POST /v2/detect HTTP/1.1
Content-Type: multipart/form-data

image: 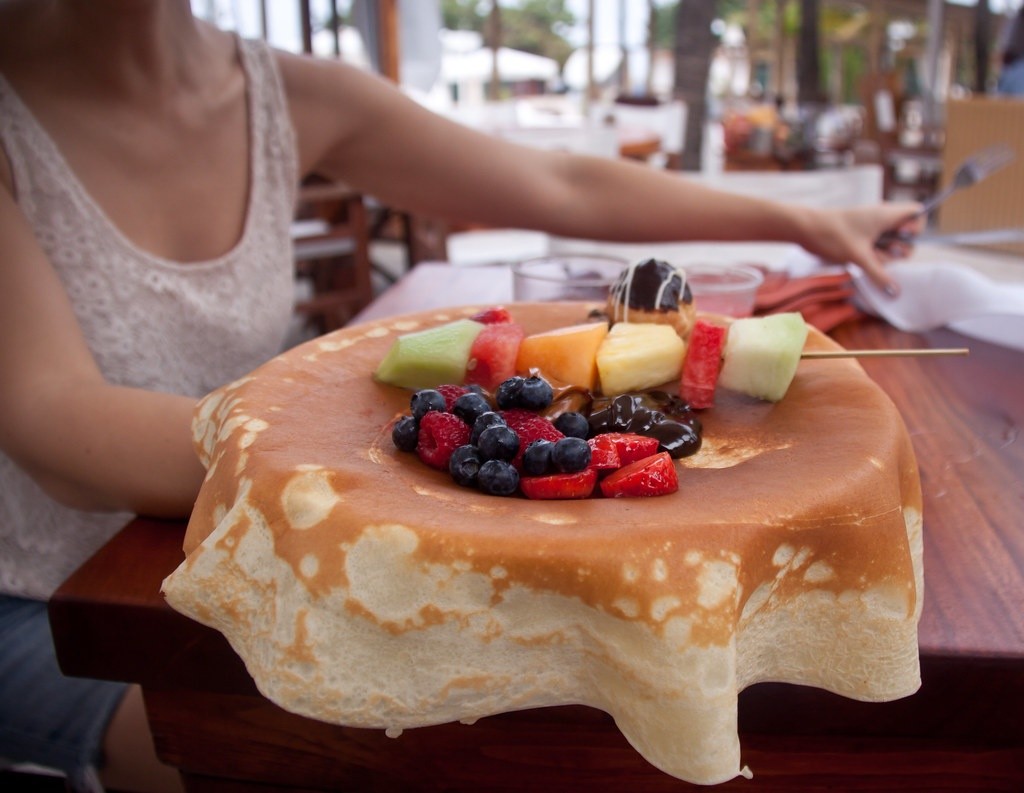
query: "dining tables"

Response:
[46,253,1024,793]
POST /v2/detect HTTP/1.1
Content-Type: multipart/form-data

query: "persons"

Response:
[2,0,930,793]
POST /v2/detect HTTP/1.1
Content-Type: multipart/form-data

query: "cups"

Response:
[510,252,635,301]
[687,260,766,319]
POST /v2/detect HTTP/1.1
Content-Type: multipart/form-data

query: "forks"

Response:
[890,152,1022,233]
